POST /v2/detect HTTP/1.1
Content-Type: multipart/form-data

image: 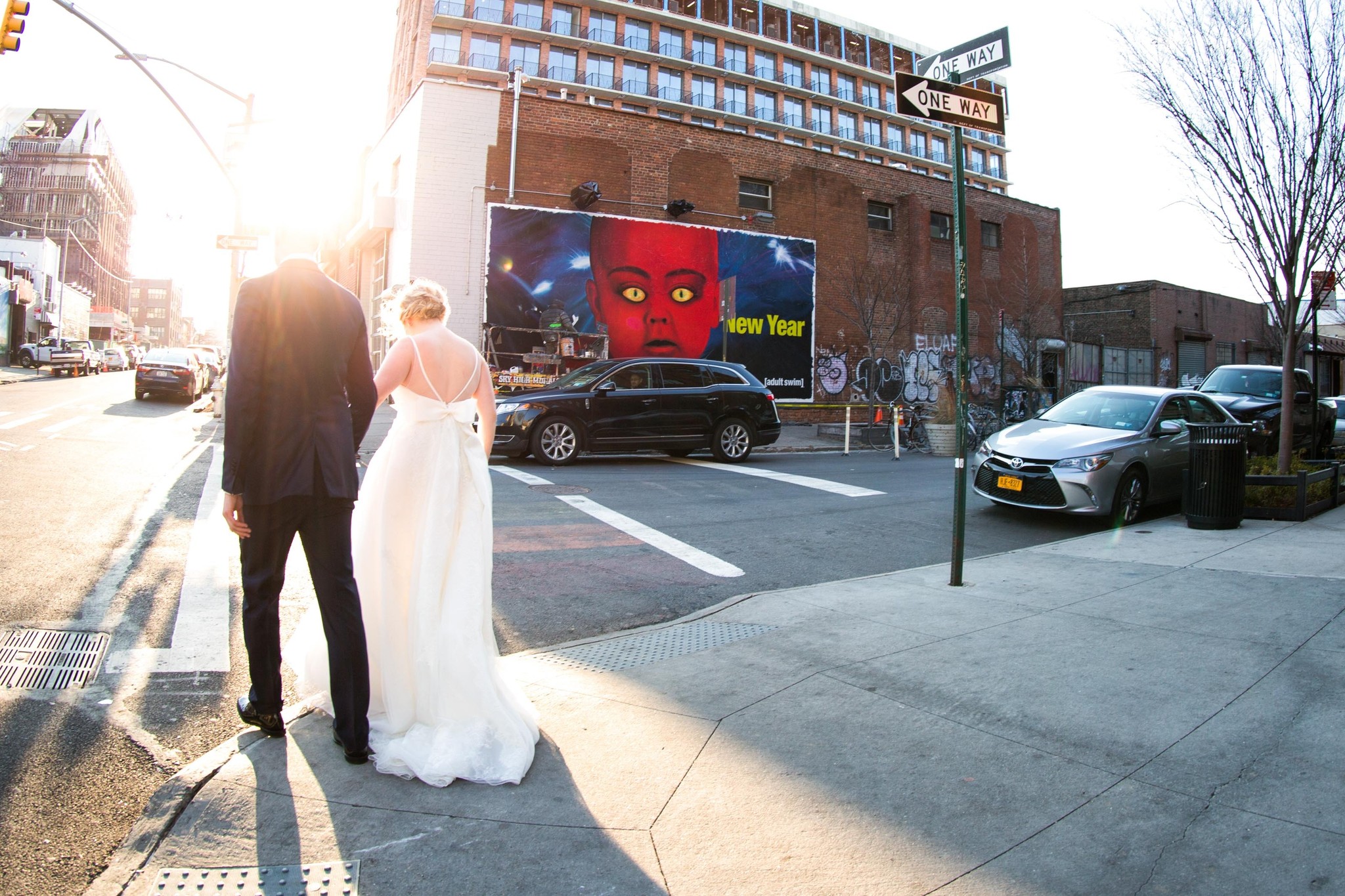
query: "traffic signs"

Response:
[894,71,1006,137]
[915,26,1012,86]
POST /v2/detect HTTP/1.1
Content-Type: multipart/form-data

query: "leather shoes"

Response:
[332,718,369,763]
[236,697,287,738]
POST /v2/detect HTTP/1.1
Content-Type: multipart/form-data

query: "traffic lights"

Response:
[0,0,30,54]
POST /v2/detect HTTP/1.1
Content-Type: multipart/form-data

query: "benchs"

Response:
[1129,399,1209,427]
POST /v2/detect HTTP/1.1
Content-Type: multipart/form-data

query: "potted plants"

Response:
[924,383,956,457]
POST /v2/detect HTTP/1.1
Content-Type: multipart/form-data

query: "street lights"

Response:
[113,52,256,349]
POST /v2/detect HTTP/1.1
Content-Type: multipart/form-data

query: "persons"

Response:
[345,280,542,786]
[585,215,723,361]
[221,258,376,771]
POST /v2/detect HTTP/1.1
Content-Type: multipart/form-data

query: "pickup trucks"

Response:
[1193,364,1337,457]
[51,340,101,376]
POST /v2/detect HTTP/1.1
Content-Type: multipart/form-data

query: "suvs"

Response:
[16,336,81,369]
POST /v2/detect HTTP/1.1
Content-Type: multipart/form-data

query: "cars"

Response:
[970,385,1248,528]
[471,357,783,464]
[186,345,227,405]
[94,341,147,373]
[135,347,205,405]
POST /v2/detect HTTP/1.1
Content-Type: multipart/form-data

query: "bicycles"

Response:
[867,400,1008,454]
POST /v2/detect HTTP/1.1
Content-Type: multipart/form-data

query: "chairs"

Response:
[1098,399,1129,429]
[1139,399,1179,429]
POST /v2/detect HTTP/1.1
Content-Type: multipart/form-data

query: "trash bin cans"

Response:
[1184,422,1253,531]
